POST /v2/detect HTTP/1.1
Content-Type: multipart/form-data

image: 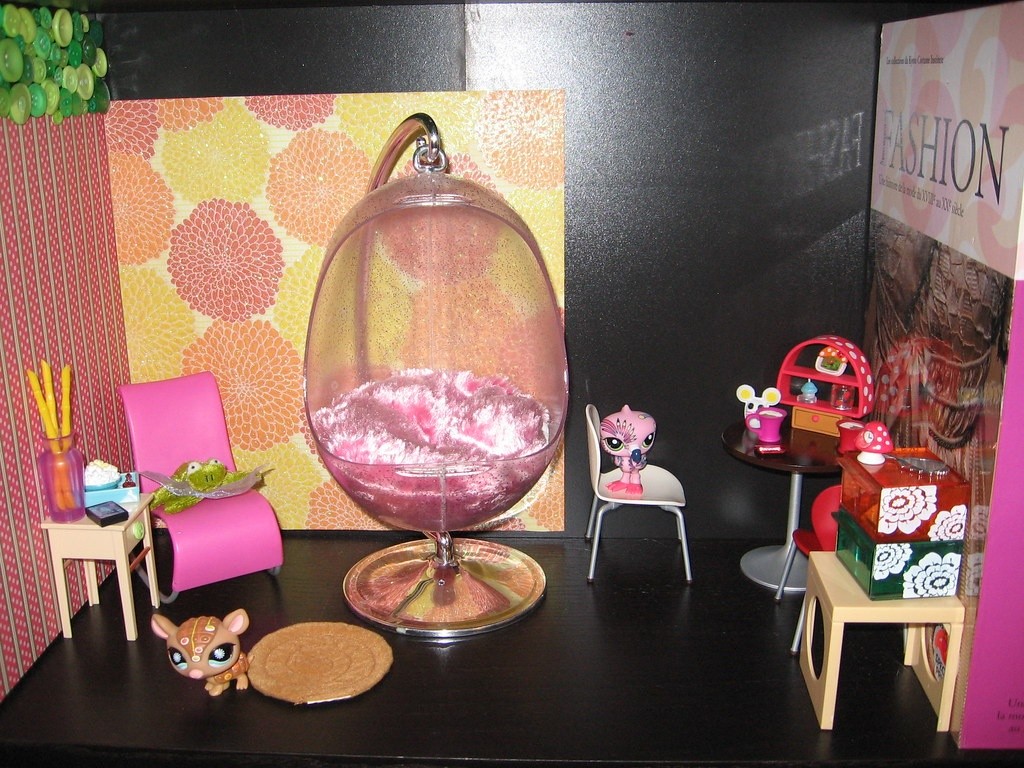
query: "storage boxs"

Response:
[790,406,851,437]
[830,446,972,601]
[82,501,129,528]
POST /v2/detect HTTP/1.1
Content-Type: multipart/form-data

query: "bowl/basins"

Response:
[83,475,122,493]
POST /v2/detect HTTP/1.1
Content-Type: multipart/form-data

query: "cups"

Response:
[745,407,788,443]
[829,383,855,411]
[835,418,867,455]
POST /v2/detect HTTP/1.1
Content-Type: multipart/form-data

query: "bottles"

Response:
[800,381,818,403]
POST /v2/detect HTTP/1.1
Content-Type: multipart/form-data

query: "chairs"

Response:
[120,370,286,605]
[774,482,844,656]
[584,405,694,585]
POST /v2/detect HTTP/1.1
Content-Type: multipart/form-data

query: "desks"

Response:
[721,420,842,593]
[39,493,160,642]
[797,550,968,733]
